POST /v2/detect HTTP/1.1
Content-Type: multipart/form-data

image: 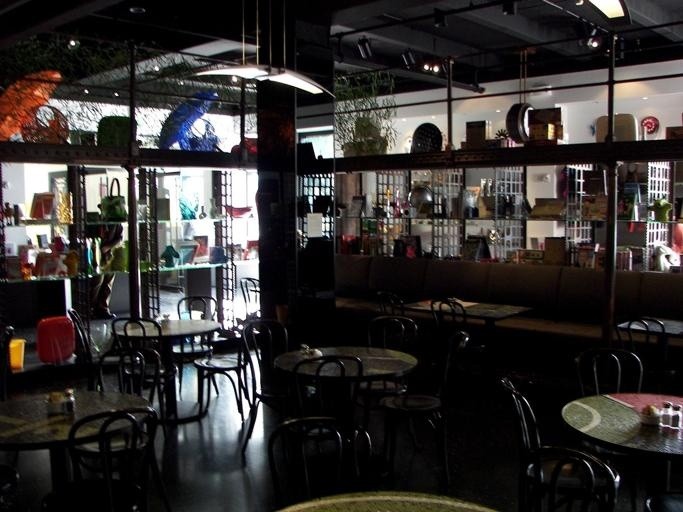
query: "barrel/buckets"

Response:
[10,337,26,371]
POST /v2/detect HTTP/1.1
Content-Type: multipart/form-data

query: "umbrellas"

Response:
[0,69,72,150]
[157,90,224,154]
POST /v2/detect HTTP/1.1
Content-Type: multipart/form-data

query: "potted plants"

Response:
[326,69,397,157]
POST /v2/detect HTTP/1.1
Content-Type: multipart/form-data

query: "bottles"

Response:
[640,401,682,430]
[48,389,76,415]
[299,344,310,361]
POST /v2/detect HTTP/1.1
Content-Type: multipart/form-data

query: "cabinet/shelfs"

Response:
[336,139,682,272]
[0,139,258,285]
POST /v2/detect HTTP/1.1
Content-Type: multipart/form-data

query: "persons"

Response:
[85,212,116,321]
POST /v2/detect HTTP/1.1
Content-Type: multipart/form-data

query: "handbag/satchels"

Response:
[101,195,128,223]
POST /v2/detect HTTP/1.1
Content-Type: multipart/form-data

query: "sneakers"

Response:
[91,308,117,321]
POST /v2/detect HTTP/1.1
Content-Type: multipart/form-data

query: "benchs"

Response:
[333,252,682,390]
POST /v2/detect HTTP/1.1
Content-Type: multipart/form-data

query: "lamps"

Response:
[190,1,336,102]
